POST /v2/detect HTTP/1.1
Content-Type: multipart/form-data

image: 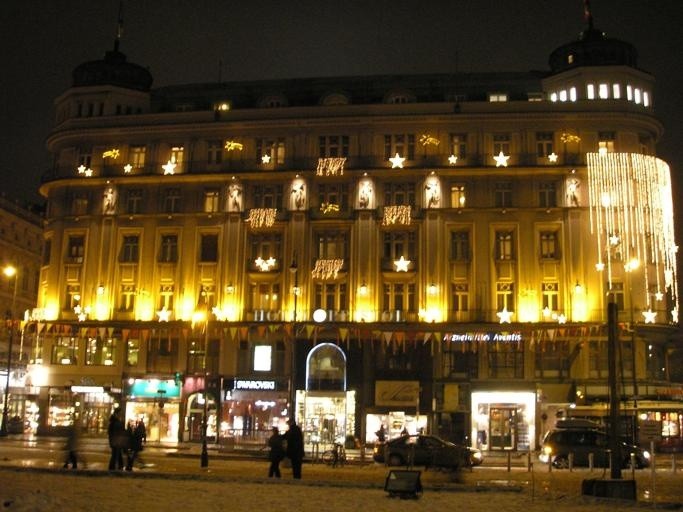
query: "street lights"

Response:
[0,256,20,437]
[195,289,211,468]
[289,248,300,421]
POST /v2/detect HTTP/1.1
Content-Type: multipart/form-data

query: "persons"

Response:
[400,427,409,437]
[108,408,147,472]
[567,177,580,208]
[360,182,371,209]
[281,419,305,479]
[61,424,77,474]
[104,188,114,214]
[291,183,305,210]
[229,184,241,210]
[267,426,286,478]
[424,180,440,208]
[376,424,386,441]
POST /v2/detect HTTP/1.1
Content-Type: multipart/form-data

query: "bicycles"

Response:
[322,441,346,467]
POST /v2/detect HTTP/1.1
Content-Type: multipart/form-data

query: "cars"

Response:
[540,418,651,470]
[373,434,483,466]
